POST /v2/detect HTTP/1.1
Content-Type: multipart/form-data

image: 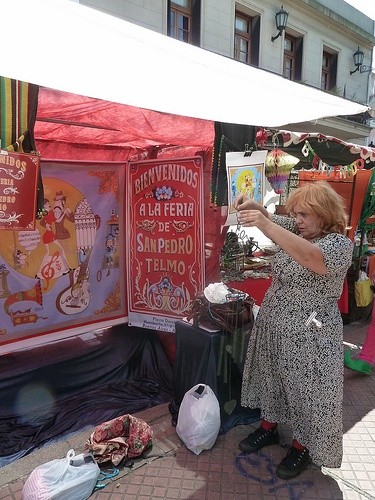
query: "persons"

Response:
[231,179,354,481]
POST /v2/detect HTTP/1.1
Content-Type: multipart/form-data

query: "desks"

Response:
[167,302,262,436]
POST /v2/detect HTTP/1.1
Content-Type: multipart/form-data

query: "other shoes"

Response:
[239,427,276,453]
[343,349,372,376]
[277,449,309,479]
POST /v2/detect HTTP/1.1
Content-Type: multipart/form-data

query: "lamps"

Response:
[271,4,288,43]
[350,46,364,75]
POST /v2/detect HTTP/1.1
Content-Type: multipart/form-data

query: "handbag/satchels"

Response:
[19,448,101,500]
[176,384,221,455]
[354,274,374,308]
